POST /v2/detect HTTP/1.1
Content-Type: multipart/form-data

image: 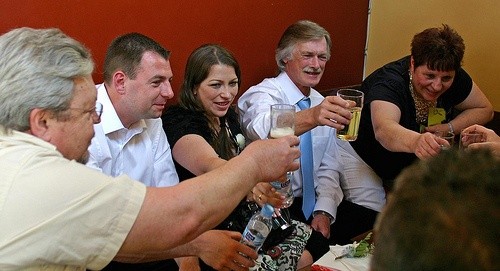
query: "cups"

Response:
[459,128,487,150]
[269,103,297,148]
[435,130,455,152]
[336,89,364,142]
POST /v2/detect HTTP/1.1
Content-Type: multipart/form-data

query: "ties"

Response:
[295,97,315,220]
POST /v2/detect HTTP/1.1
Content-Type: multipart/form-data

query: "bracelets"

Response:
[447,122,455,139]
[313,210,334,224]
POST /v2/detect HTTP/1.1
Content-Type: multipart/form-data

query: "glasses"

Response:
[56,102,103,121]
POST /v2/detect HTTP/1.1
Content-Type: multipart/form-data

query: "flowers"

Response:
[336,233,378,260]
[236,132,246,152]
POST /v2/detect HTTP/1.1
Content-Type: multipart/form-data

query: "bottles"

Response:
[233,204,274,267]
[270,171,295,209]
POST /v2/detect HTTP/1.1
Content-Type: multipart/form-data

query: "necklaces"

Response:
[408,78,437,124]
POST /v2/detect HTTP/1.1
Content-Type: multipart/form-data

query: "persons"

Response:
[162,42,330,271]
[460,123,499,161]
[0,25,301,271]
[368,147,500,271]
[349,24,495,236]
[82,32,258,271]
[232,19,345,264]
[328,136,387,253]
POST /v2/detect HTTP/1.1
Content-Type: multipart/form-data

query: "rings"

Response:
[258,193,264,202]
